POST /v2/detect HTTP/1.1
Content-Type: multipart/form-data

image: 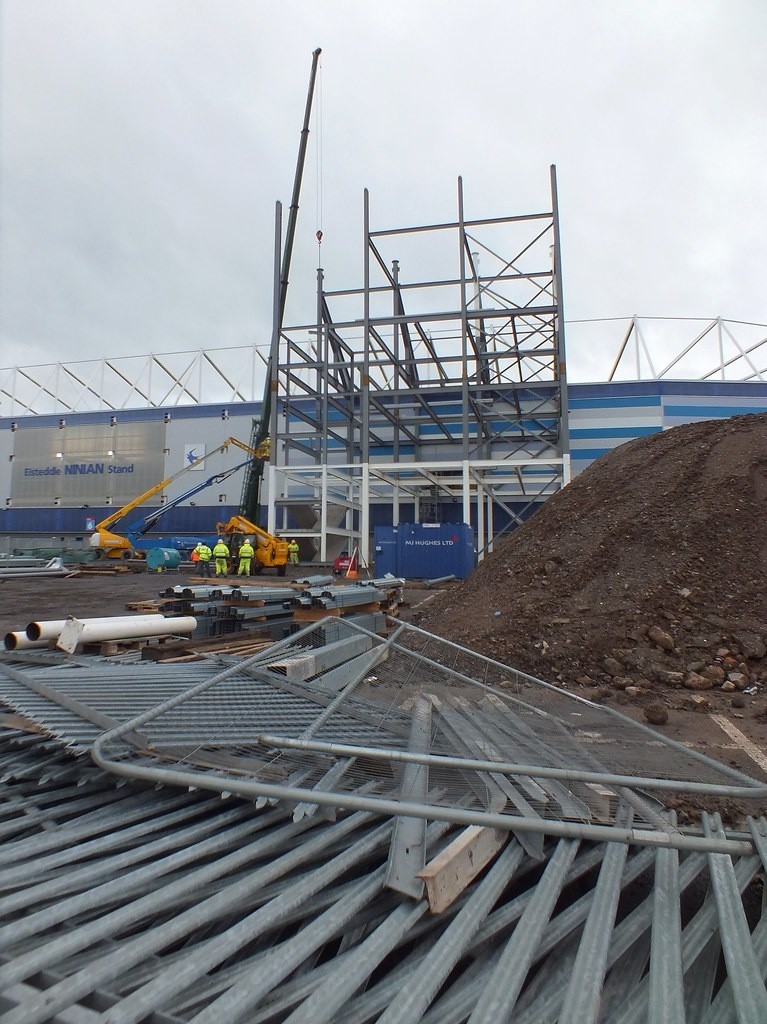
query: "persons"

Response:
[213,539,229,578]
[236,539,254,578]
[262,437,270,457]
[288,540,300,564]
[191,542,212,578]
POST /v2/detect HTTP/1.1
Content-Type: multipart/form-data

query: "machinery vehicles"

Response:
[126,457,256,556]
[86,437,269,560]
[217,514,289,577]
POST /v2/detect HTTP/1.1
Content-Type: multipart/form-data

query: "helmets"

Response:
[202,543,206,545]
[245,539,250,543]
[291,540,295,544]
[218,539,223,543]
[197,542,202,546]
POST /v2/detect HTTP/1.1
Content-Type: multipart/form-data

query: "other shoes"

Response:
[296,566,299,567]
[201,576,204,578]
[215,576,220,578]
[208,576,212,578]
[224,575,227,578]
[246,576,250,578]
[236,576,241,578]
[292,566,295,568]
